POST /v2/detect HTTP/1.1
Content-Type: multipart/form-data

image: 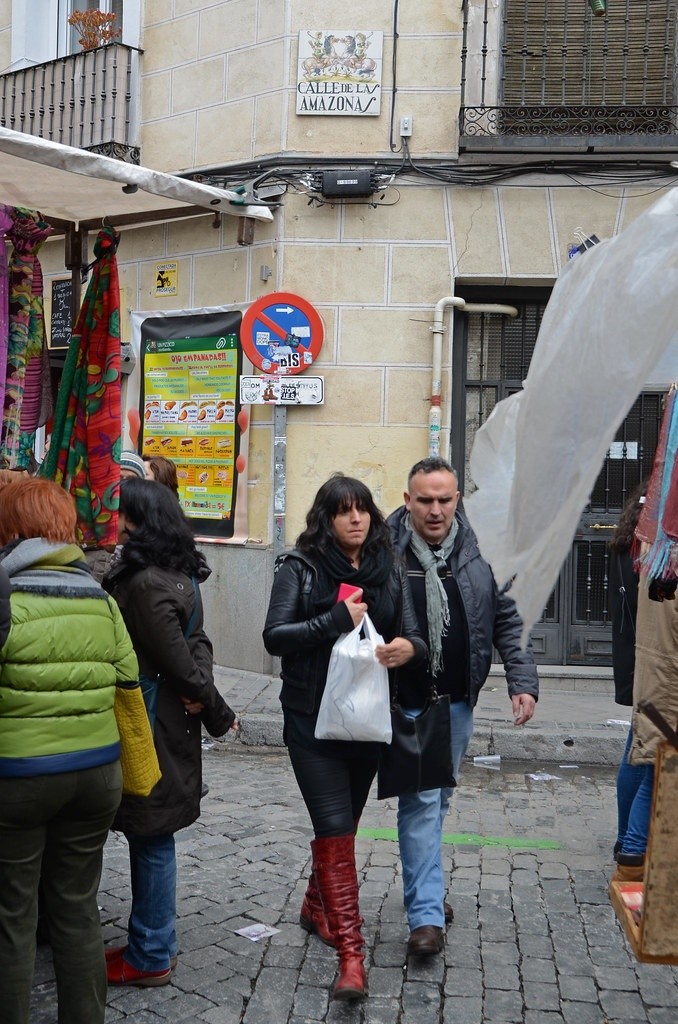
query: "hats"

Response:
[119,450,146,478]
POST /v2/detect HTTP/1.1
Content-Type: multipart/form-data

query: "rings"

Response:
[388,657,392,662]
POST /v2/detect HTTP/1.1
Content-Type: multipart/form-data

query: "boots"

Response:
[310,836,364,997]
[300,874,336,948]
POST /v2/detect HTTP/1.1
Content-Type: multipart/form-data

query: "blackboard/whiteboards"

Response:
[47,278,73,350]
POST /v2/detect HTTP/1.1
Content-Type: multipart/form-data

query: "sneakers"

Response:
[105,948,177,969]
[107,956,172,986]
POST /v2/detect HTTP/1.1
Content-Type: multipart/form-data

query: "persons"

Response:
[120,449,180,500]
[262,476,430,1000]
[609,488,653,880]
[378,457,538,960]
[100,479,237,984]
[0,478,138,1024]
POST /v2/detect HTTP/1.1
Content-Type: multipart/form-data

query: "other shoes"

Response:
[613,841,623,860]
[616,855,646,882]
[202,783,209,798]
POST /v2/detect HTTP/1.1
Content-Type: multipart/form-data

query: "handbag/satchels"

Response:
[114,681,162,796]
[140,682,157,727]
[314,613,392,744]
[378,664,456,800]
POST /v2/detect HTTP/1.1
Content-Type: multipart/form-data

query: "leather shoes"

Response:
[443,902,453,922]
[408,925,444,956]
